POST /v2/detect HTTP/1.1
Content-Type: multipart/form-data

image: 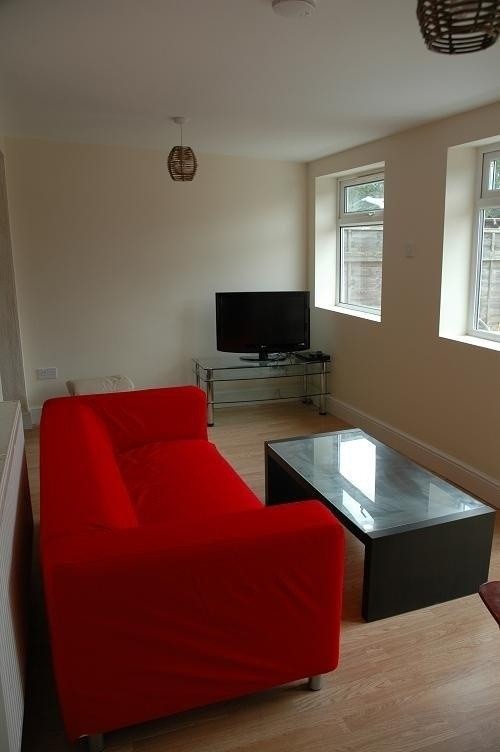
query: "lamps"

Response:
[169,118,196,182]
[417,0,500,54]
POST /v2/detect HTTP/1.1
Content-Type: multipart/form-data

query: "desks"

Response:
[195,360,334,426]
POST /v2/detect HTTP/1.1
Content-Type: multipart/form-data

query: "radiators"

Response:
[0,401,33,752]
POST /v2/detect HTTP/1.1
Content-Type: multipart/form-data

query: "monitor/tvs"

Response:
[214,290,311,362]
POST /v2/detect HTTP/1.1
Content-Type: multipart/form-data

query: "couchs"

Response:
[38,386,344,751]
